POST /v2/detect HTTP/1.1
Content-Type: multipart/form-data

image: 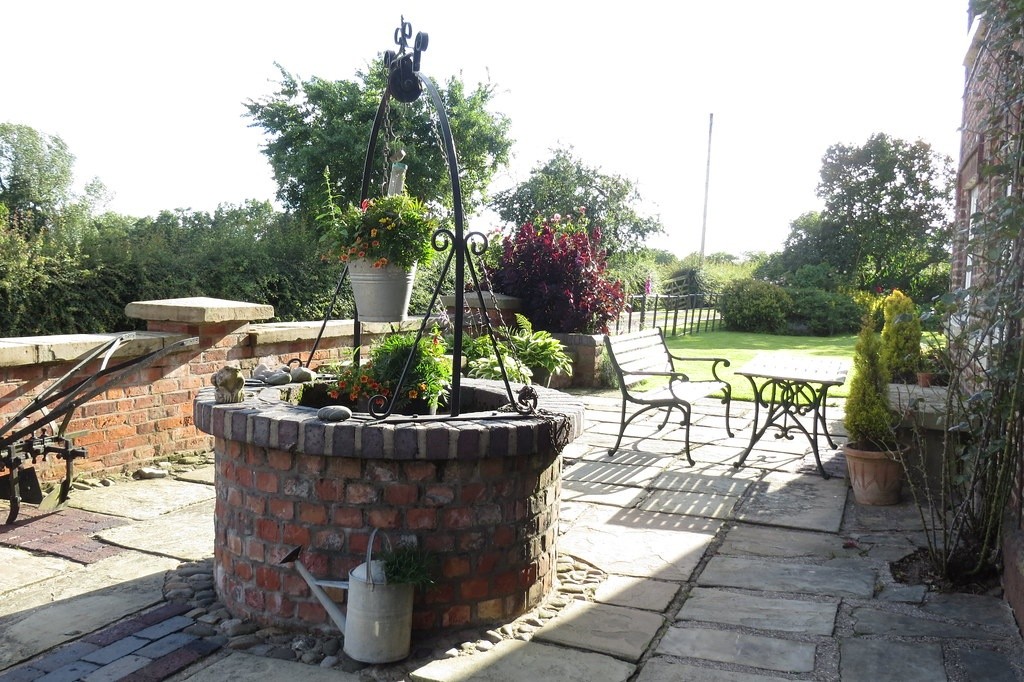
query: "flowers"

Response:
[318,320,453,408]
[314,165,439,273]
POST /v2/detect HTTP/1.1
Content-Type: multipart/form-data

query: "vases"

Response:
[356,396,438,415]
[345,256,417,323]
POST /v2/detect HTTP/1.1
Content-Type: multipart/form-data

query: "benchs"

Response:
[604,326,734,466]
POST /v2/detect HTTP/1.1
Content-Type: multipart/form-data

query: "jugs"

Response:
[278,527,414,663]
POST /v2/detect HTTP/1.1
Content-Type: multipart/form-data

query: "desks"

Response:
[734,350,852,480]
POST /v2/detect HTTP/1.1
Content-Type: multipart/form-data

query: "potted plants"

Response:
[444,314,576,388]
[911,351,933,387]
[843,312,911,506]
